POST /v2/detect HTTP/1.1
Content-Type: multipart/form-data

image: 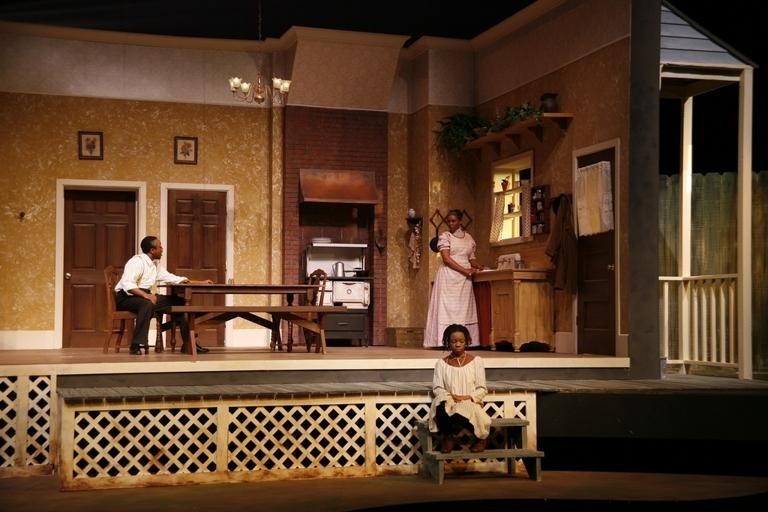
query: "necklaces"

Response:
[447,353,466,367]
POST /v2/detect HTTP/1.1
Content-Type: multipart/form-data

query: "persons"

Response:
[424,210,483,348]
[429,324,492,453]
[113,237,214,354]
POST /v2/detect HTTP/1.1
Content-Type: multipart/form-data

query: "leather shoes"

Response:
[180,344,208,354]
[129,345,142,355]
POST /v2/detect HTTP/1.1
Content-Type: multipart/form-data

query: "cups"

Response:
[508,203,515,214]
[502,179,509,192]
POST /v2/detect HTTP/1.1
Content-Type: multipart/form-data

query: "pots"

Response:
[353,267,369,277]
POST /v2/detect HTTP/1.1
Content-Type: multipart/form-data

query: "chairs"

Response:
[269,269,326,354]
[103,266,155,355]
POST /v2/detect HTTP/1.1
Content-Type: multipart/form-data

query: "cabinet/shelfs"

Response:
[306,242,374,346]
[529,184,551,236]
[489,150,534,247]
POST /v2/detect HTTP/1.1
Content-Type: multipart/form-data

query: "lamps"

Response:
[228,1,292,103]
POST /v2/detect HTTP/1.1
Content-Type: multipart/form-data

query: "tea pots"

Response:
[331,260,345,277]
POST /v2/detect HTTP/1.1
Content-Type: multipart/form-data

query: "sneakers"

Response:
[469,432,488,452]
[439,433,452,453]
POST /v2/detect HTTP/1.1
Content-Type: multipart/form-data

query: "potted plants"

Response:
[501,175,510,192]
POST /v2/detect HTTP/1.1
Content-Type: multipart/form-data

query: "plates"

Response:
[311,238,332,243]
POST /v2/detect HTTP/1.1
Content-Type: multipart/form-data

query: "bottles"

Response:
[539,92,557,114]
[532,189,545,233]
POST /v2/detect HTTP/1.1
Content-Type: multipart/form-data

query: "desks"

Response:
[473,268,556,353]
[157,283,324,356]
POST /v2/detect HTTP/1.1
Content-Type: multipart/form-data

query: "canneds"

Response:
[532,189,543,233]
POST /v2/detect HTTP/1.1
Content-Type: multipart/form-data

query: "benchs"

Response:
[414,419,545,483]
[166,306,348,314]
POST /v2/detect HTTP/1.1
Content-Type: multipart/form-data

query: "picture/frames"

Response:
[174,136,198,164]
[78,130,103,160]
